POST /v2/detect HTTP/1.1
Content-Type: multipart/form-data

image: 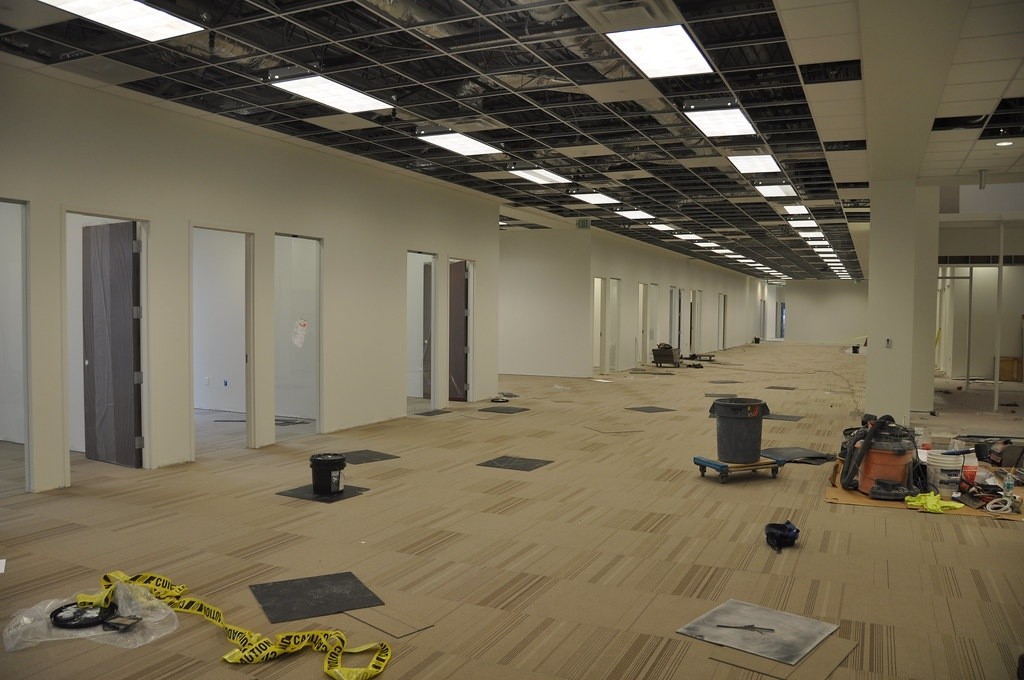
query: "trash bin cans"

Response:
[708,398,770,464]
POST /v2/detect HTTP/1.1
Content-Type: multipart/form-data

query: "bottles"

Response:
[1003,473,1014,500]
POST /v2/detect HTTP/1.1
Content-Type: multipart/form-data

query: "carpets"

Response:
[625,366,804,422]
[479,406,530,413]
[760,446,838,466]
[277,482,370,504]
[342,449,401,465]
[249,571,385,624]
[476,454,555,473]
[413,409,452,416]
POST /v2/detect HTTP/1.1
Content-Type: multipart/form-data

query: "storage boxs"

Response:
[994,356,1024,382]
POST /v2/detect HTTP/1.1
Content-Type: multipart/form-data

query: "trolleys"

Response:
[693,450,787,482]
[651,348,683,368]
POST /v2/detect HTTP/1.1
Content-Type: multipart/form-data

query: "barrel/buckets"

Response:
[927,450,964,498]
[310,453,346,496]
[755,338,760,343]
[852,346,859,353]
